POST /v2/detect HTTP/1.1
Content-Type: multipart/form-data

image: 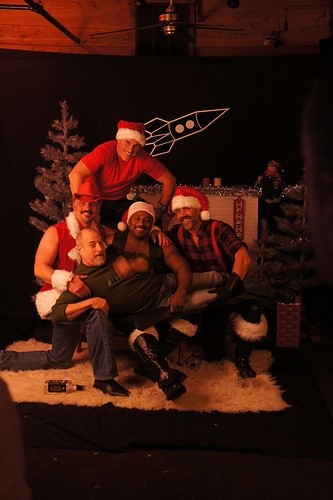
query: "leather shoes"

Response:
[94,379,131,396]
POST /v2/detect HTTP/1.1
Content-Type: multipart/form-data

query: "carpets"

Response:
[0,338,291,413]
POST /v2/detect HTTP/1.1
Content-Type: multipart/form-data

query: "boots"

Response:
[225,312,268,378]
[134,327,186,400]
[133,333,188,388]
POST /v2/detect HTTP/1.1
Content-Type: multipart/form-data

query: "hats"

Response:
[172,186,210,220]
[72,176,104,202]
[116,120,146,147]
[117,202,156,231]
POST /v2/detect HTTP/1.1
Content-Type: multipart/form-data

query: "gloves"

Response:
[208,272,245,314]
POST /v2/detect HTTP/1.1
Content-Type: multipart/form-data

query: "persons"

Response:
[50,228,299,326]
[0,179,130,395]
[96,203,200,399]
[66,120,176,230]
[151,184,296,378]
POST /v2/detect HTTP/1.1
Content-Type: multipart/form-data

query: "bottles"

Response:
[43,379,87,395]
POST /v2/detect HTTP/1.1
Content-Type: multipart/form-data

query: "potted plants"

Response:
[248,184,317,347]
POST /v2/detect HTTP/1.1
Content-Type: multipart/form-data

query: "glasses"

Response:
[121,139,142,152]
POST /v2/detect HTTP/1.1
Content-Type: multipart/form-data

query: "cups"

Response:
[203,178,210,187]
[214,178,221,187]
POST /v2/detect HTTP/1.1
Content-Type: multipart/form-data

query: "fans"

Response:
[88,0,244,42]
